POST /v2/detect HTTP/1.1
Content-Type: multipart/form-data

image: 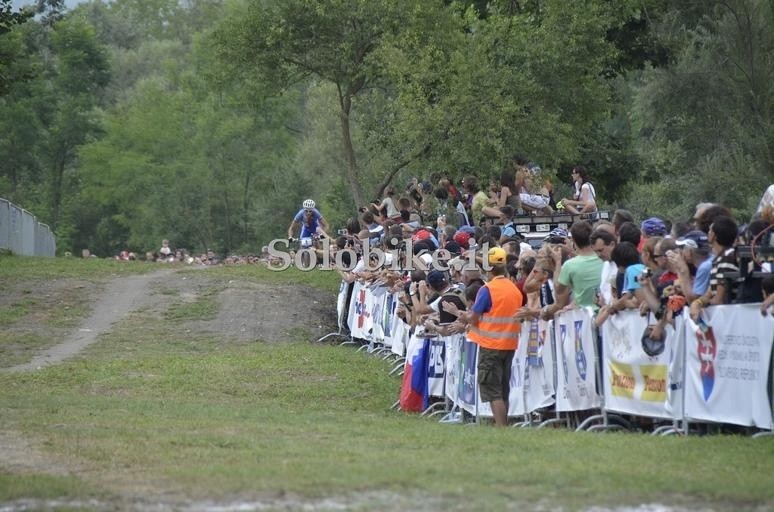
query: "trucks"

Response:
[477,211,610,249]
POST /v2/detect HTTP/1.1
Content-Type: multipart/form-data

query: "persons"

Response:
[458,246,524,427]
[81,238,303,267]
[288,199,329,248]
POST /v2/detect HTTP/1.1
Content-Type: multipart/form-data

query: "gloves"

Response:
[288,237,293,243]
[320,234,324,239]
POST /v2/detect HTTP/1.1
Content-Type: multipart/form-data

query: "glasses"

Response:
[305,210,312,213]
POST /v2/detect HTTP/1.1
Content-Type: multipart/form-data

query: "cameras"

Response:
[641,267,654,278]
[370,196,380,205]
[358,206,366,212]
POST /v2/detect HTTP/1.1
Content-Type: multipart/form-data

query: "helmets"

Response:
[302,199,316,209]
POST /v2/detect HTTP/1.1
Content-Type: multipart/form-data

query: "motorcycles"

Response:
[286,234,325,264]
[153,251,177,263]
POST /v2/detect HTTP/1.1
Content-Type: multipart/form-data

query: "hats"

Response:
[640,218,667,237]
[674,231,708,250]
[410,228,507,284]
